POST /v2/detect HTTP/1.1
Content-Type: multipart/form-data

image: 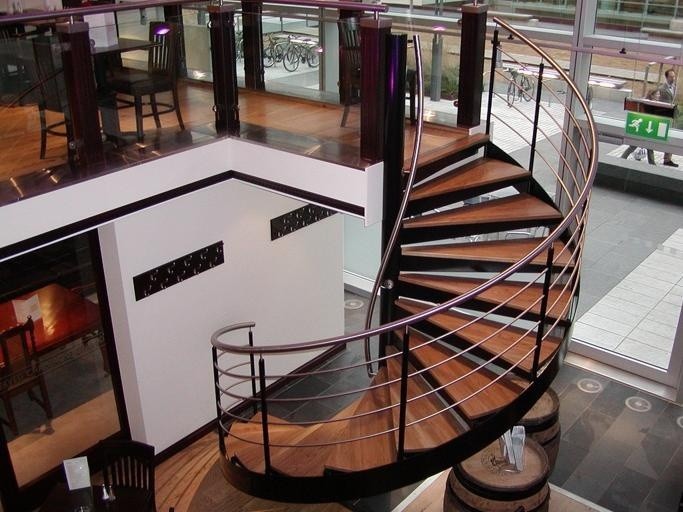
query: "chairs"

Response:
[1,7,186,168]
[332,15,418,126]
[97,437,158,511]
[0,314,53,437]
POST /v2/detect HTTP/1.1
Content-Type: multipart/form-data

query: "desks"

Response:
[0,478,153,511]
[0,284,112,390]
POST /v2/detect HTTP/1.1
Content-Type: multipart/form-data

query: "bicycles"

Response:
[235,28,320,73]
[504,65,535,107]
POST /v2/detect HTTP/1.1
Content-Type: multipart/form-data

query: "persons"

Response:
[655,68,680,167]
[621,89,661,167]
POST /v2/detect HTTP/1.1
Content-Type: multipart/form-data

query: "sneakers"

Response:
[663,161,679,168]
